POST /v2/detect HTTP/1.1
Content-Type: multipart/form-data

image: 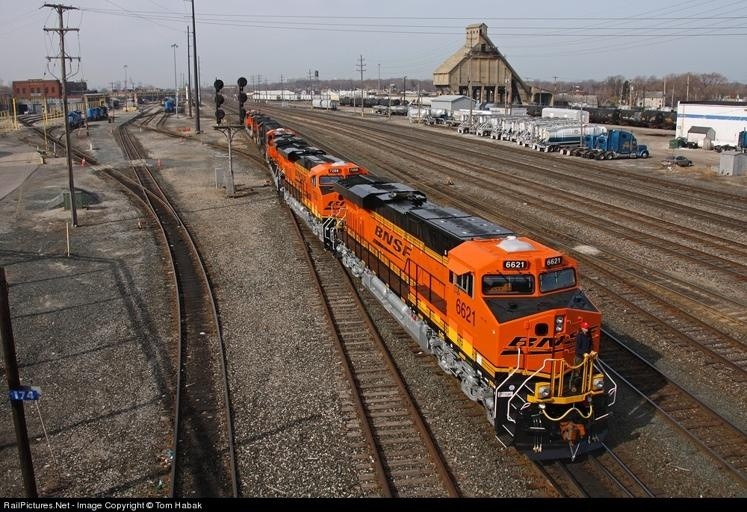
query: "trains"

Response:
[239,105,618,464]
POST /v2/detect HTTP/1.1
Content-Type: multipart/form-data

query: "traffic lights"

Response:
[236,76,248,124]
[213,78,225,124]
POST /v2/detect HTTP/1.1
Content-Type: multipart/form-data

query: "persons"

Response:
[569,322,593,393]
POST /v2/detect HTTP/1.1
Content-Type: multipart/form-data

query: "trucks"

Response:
[455,111,649,161]
